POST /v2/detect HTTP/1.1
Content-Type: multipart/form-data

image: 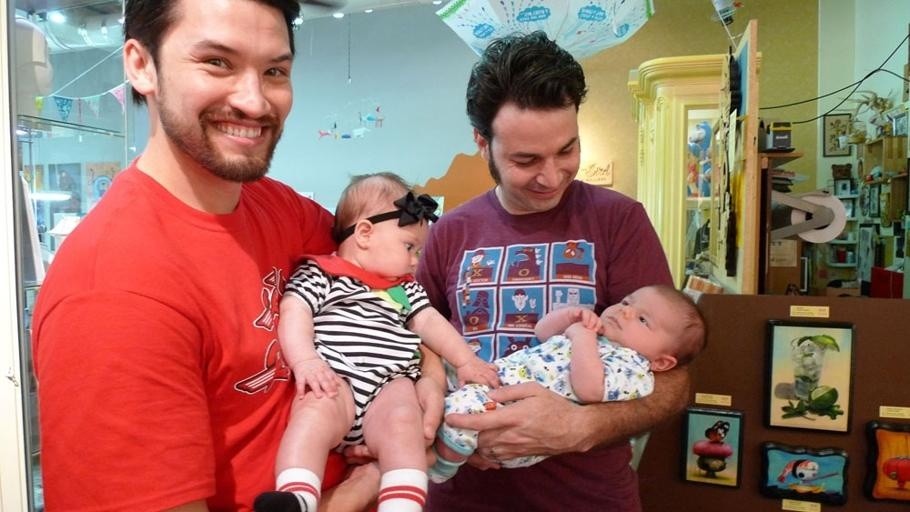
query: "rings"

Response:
[490,446,497,459]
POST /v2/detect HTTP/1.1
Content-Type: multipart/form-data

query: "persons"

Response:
[417,29,693,512]
[425,283,709,487]
[27,0,449,512]
[251,169,503,511]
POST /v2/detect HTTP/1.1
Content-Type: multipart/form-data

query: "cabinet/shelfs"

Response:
[826,193,859,268]
[864,135,909,220]
[625,52,762,299]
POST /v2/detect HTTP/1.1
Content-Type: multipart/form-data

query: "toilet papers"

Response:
[772,190,848,244]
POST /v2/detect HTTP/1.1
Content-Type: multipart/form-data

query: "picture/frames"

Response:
[758,318,857,507]
[833,179,852,196]
[868,184,881,217]
[893,110,908,136]
[823,113,852,158]
[679,406,745,489]
[860,421,910,504]
